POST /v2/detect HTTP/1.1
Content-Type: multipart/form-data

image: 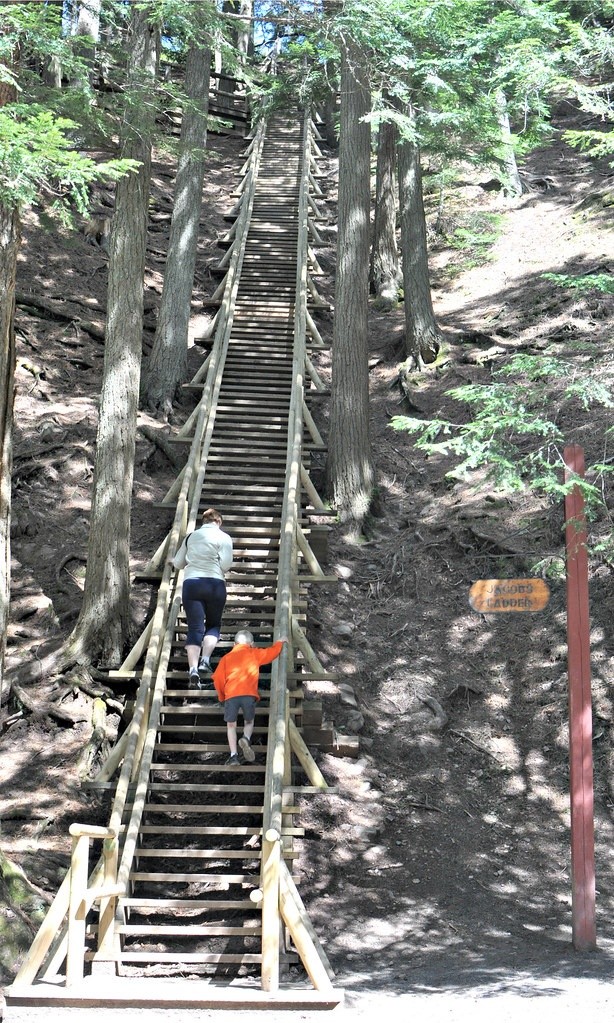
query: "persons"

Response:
[210,630,289,767]
[173,508,233,689]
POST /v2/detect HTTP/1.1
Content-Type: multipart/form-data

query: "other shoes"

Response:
[238,736,255,762]
[225,753,240,766]
[198,660,214,673]
[188,667,202,689]
